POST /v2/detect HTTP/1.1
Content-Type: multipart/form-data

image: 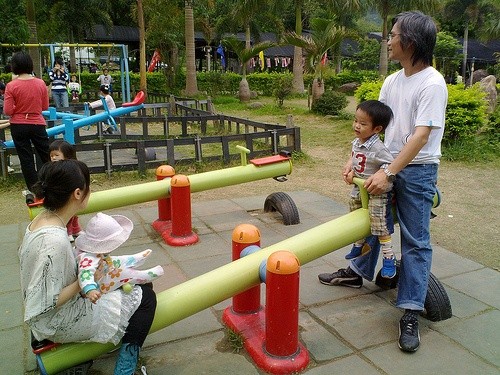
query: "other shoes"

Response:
[68,235,74,241]
[72,231,84,236]
[96,130,109,135]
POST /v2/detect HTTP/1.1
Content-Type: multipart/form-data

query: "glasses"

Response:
[389,33,401,40]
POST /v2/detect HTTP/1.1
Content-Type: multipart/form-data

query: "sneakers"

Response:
[381,253,396,279]
[318,268,363,288]
[397,318,421,351]
[345,245,363,261]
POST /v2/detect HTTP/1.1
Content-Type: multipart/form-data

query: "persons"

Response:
[68,75,80,101]
[17,160,157,375]
[49,60,70,113]
[49,139,85,242]
[97,69,116,97]
[89,63,97,73]
[73,212,164,304]
[3,52,50,193]
[318,11,448,352]
[88,85,116,135]
[345,100,397,278]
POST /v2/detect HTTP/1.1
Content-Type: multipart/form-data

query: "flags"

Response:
[216,45,225,68]
[259,51,264,71]
[147,51,161,73]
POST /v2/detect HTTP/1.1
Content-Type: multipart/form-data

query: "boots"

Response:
[114,342,140,375]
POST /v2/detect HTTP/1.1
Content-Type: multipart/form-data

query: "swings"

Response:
[61,47,82,94]
[97,44,113,93]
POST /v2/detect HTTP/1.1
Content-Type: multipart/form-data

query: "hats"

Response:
[75,212,133,253]
